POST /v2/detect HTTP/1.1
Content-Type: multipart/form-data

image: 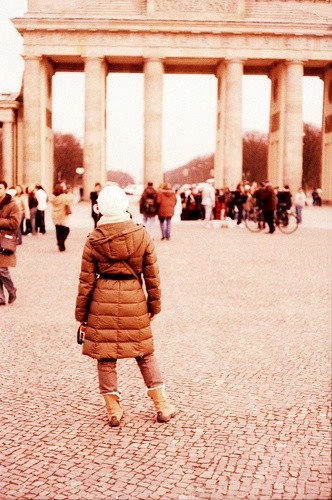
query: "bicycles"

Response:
[245,202,299,235]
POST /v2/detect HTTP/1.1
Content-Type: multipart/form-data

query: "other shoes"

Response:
[0,303,6,305]
[8,287,17,304]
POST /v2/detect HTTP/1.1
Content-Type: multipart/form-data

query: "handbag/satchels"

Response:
[0,233,18,254]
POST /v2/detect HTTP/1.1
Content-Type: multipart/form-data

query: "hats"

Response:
[96,185,129,216]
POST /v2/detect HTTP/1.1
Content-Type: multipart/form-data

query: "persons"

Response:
[139,181,158,240]
[172,177,323,234]
[75,184,180,425]
[157,183,177,240]
[25,184,48,238]
[90,182,103,229]
[1,180,21,305]
[51,182,73,252]
[79,185,84,201]
[13,185,31,245]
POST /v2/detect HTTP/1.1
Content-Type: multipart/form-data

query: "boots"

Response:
[103,391,125,427]
[147,384,180,423]
